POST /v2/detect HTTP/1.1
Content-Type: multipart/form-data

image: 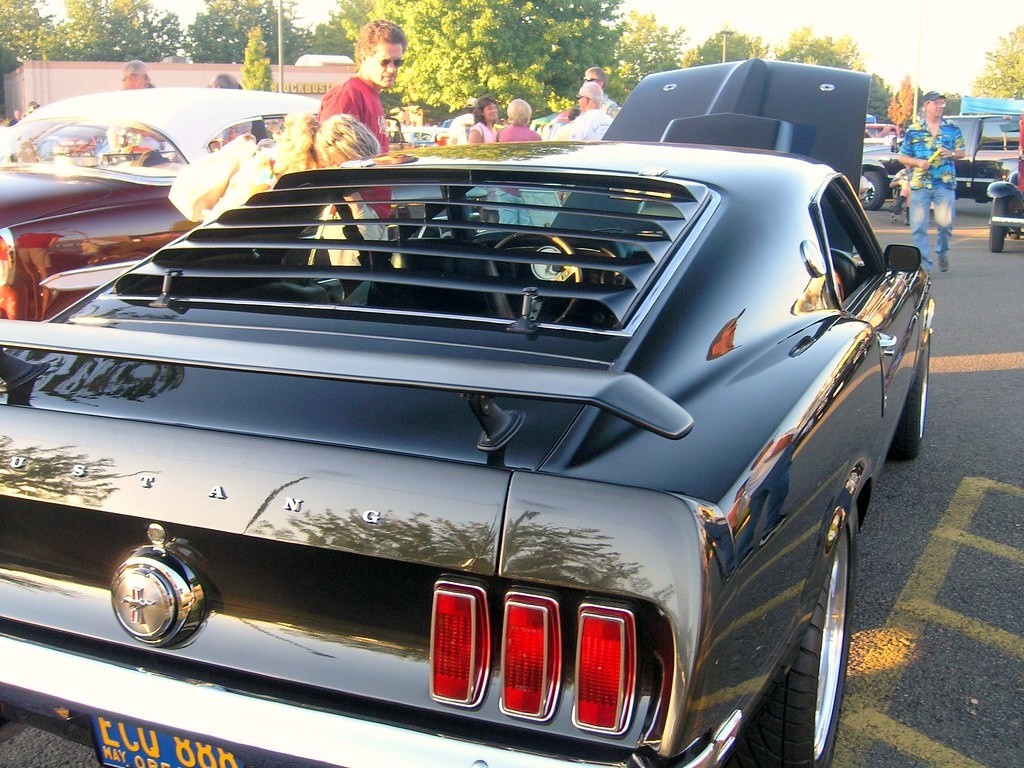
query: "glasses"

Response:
[576,96,582,100]
[583,79,598,83]
[33,105,40,109]
[379,59,404,67]
[130,73,152,81]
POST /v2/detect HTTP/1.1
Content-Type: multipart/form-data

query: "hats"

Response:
[922,91,946,102]
[466,98,478,108]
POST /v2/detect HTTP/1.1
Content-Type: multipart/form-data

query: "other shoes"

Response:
[938,257,949,272]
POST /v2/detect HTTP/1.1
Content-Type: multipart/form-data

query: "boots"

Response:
[904,207,909,225]
[888,196,906,215]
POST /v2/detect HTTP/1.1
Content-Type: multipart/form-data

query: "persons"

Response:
[889,91,966,278]
[0,19,622,268]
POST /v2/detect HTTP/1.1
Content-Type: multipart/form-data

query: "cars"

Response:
[0,58,935,768]
[0,89,325,325]
[863,125,899,138]
[440,119,454,128]
[986,158,1024,253]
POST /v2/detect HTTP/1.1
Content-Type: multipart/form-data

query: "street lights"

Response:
[721,31,734,61]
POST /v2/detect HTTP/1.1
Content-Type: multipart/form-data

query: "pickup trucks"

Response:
[385,117,436,153]
[858,114,1021,211]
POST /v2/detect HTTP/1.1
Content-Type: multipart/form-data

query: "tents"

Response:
[960,97,1024,115]
